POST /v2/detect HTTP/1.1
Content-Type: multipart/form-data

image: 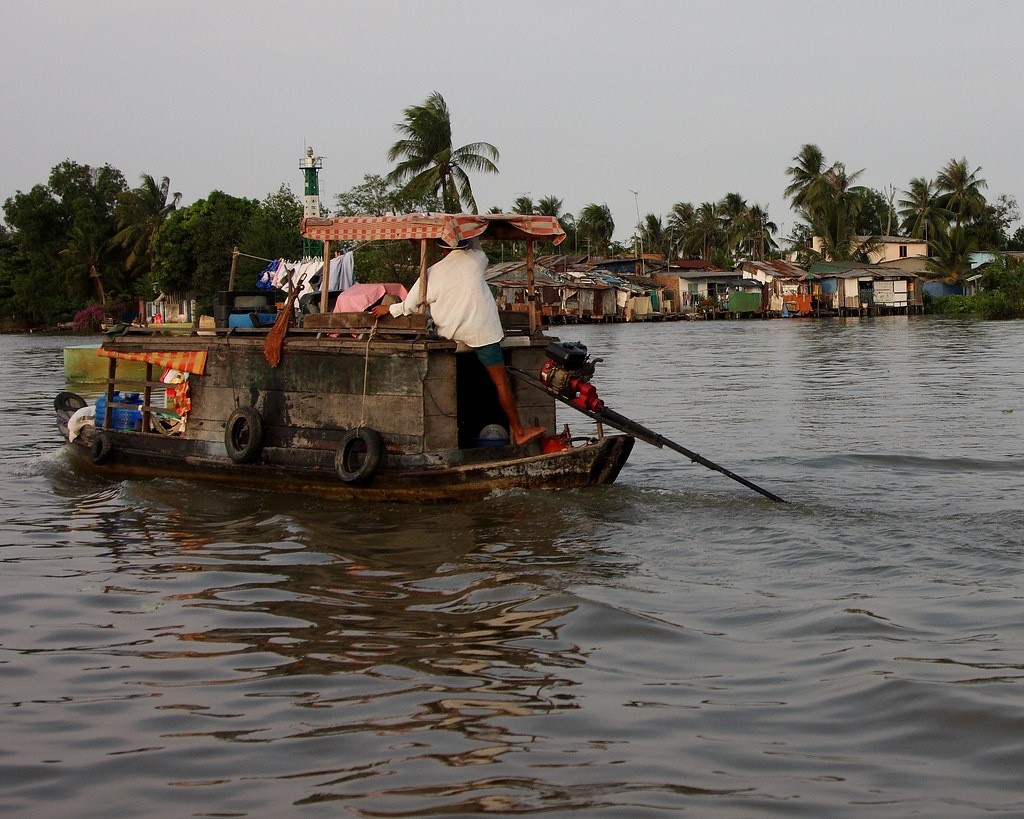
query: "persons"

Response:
[371,236,548,446]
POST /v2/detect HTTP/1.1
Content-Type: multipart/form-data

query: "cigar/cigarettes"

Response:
[368,312,372,314]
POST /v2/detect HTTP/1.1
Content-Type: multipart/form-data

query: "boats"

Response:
[49,140,637,503]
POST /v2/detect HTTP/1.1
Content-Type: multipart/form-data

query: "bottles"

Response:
[115,392,144,432]
[95,390,123,428]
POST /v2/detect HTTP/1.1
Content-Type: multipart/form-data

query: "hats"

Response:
[436,237,471,248]
